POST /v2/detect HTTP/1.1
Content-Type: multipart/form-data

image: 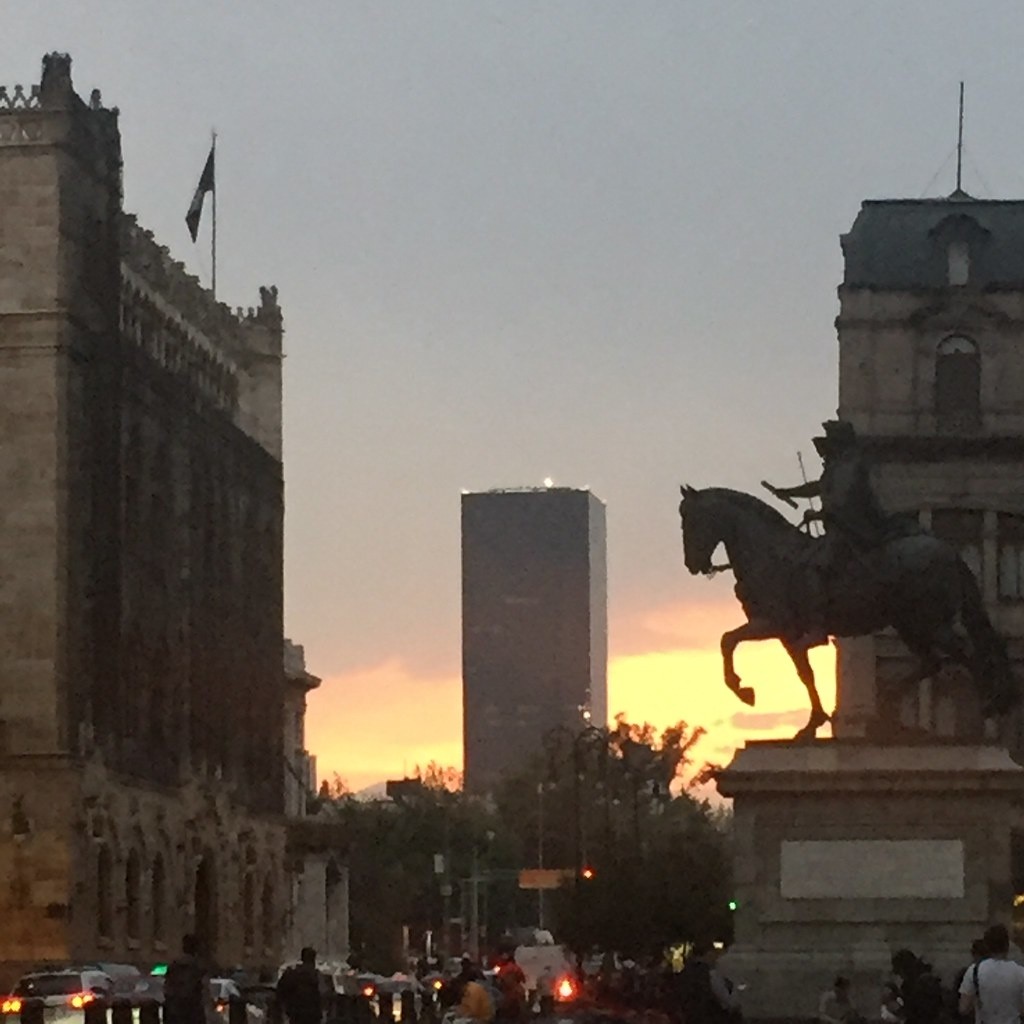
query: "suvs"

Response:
[4,967,121,1024]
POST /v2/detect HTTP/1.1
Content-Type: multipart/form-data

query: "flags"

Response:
[185,147,216,244]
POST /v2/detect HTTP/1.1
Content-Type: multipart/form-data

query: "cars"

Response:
[126,979,267,1024]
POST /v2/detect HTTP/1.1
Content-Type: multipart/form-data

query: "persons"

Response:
[162,924,1024,1024]
[773,421,886,649]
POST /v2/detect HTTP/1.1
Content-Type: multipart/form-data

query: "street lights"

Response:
[543,724,616,980]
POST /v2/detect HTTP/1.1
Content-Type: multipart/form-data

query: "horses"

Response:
[677,482,1016,739]
